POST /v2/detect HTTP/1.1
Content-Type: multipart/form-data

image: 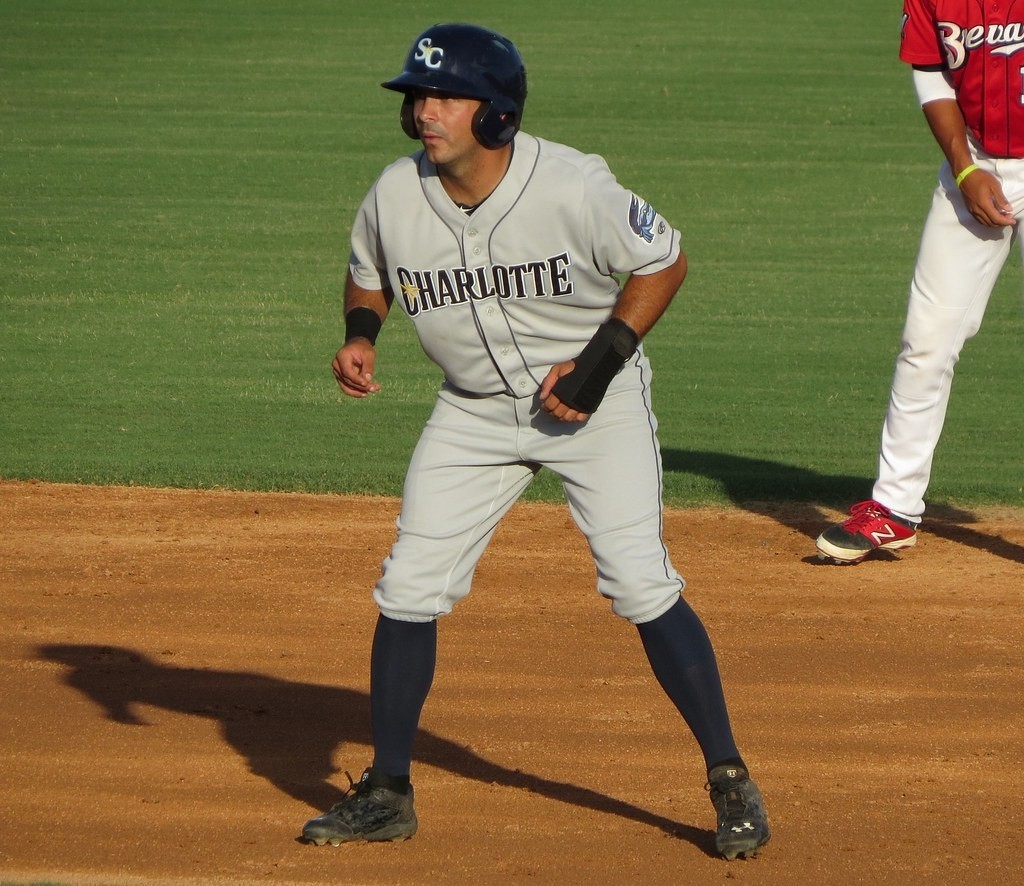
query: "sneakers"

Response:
[811,500,917,565]
[704,761,772,859]
[299,762,418,844]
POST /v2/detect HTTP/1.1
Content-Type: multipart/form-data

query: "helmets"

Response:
[381,21,529,149]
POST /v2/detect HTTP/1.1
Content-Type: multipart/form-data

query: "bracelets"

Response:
[955,163,979,188]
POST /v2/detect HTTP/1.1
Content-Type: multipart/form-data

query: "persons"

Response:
[812,2,1020,567]
[299,24,768,867]
